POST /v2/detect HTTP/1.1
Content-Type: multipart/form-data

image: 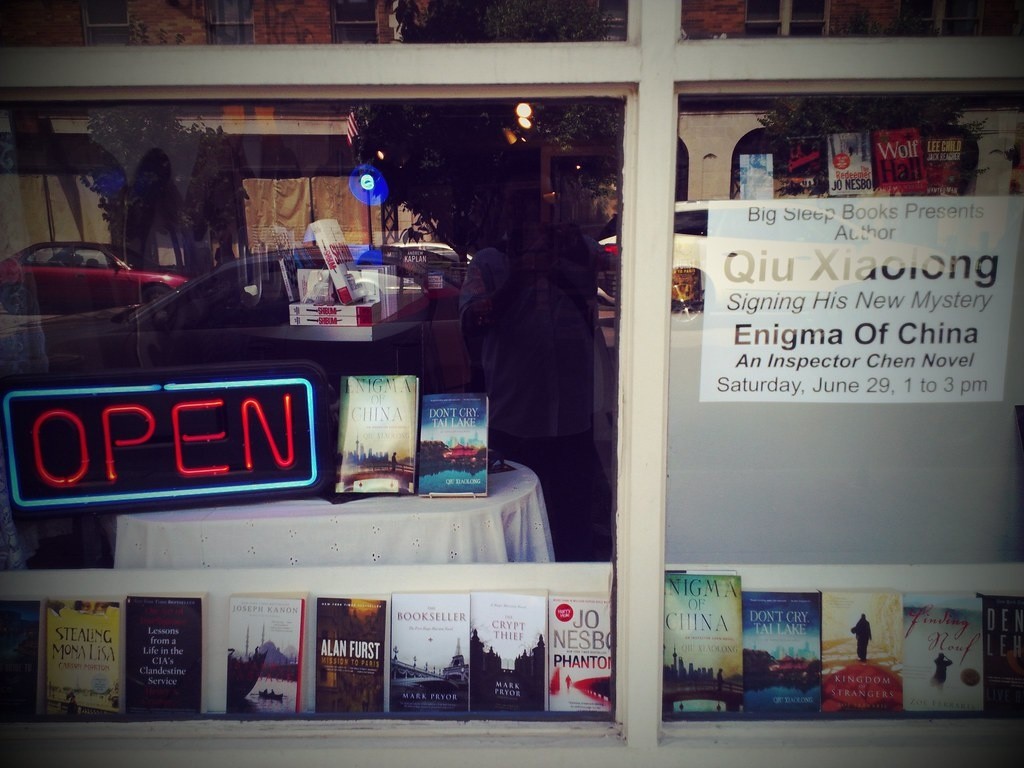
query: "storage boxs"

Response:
[271,223,399,326]
[403,272,443,289]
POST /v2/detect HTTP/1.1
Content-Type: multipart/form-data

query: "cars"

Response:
[1,242,468,361]
[1,241,191,311]
[388,241,461,264]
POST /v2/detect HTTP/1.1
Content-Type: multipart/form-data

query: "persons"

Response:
[457,187,614,470]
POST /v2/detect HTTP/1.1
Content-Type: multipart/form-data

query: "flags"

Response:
[345,104,360,146]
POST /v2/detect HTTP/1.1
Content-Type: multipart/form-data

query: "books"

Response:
[0,567,1024,712]
[337,371,420,497]
[286,219,468,328]
[420,392,490,497]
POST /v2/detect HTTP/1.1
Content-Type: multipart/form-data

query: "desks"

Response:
[112,455,555,571]
[245,321,428,375]
